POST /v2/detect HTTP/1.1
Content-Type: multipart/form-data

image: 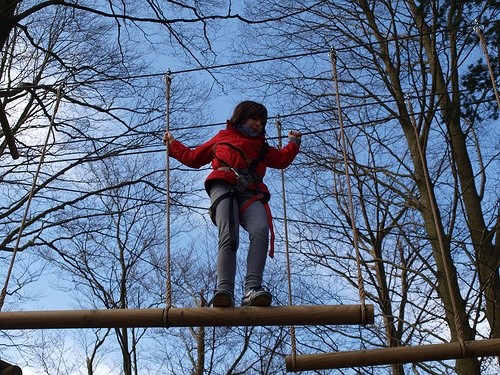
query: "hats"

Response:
[231,101,267,127]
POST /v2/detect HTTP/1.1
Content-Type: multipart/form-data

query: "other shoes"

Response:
[213,290,234,308]
[241,289,272,307]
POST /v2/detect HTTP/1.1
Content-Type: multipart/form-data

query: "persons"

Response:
[162,100,303,308]
